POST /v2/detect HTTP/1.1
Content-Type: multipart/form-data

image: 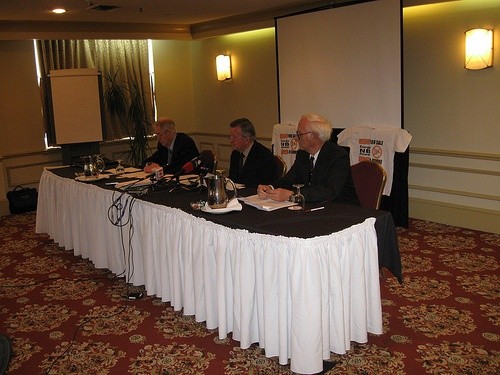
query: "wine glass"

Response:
[116,160,125,176]
[290,184,306,211]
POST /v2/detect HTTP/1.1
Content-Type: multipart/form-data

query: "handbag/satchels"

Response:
[6,186,38,214]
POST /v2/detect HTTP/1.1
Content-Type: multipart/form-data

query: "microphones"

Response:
[166,156,202,182]
[139,165,174,181]
[196,163,211,181]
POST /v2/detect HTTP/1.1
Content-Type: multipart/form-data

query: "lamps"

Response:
[464,27,494,71]
[215,54,232,82]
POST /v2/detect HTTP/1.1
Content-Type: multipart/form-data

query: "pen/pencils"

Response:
[304,206,325,213]
[169,187,176,193]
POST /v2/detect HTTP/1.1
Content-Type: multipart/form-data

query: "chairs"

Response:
[351,160,387,210]
[200,149,218,175]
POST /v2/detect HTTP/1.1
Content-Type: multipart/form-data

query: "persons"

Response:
[227,118,284,187]
[257,113,361,207]
[141,118,201,177]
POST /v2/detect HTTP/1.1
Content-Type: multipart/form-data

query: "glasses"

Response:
[296,131,312,140]
[155,129,169,138]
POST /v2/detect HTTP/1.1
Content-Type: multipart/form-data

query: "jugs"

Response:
[201,175,237,210]
[80,155,105,180]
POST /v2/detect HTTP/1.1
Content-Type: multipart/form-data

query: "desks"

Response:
[35,161,402,374]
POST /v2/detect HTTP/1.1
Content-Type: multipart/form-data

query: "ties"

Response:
[240,152,245,168]
[309,156,315,171]
